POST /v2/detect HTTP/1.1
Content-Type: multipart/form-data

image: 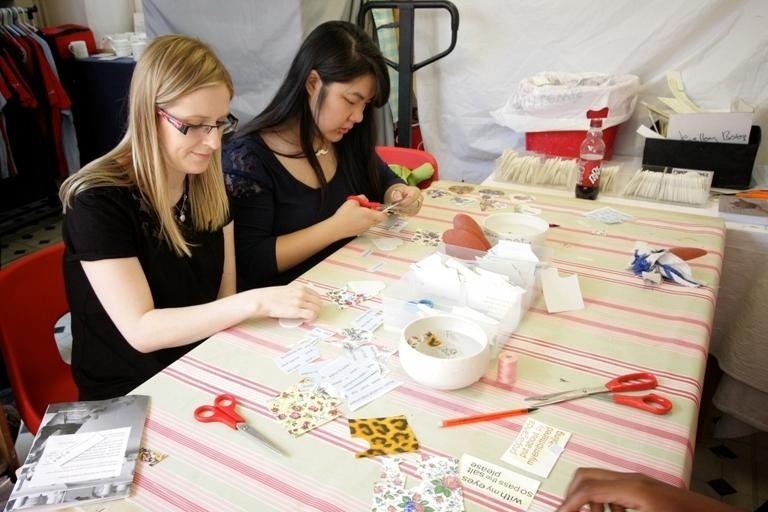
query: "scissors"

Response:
[347,195,402,222]
[523,370,673,415]
[194,394,290,459]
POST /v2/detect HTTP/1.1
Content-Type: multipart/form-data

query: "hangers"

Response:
[1,4,44,39]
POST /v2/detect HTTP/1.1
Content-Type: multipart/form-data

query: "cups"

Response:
[111,40,131,59]
[68,40,89,59]
[131,42,147,63]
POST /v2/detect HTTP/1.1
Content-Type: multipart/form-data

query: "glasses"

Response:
[152,104,238,137]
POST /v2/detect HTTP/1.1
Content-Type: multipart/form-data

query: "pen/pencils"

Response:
[442,406,540,428]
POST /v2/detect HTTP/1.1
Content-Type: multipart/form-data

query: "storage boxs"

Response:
[376,242,550,361]
[655,96,756,142]
[639,118,762,192]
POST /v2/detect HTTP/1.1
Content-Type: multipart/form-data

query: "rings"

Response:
[416,197,422,207]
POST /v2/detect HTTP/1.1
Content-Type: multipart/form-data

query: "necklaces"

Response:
[168,178,188,222]
[313,138,331,156]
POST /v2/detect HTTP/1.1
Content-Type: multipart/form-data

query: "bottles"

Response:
[574,118,607,201]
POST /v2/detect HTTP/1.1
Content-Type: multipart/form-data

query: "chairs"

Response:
[375,143,440,192]
[1,240,85,436]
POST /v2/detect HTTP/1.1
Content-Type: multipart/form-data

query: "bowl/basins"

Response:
[399,314,493,393]
[482,211,551,249]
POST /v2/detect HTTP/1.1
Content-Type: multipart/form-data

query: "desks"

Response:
[58,46,137,170]
[480,154,766,448]
[23,176,726,512]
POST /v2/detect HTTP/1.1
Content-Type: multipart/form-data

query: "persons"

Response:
[553,466,768,512]
[222,21,424,291]
[57,34,323,402]
